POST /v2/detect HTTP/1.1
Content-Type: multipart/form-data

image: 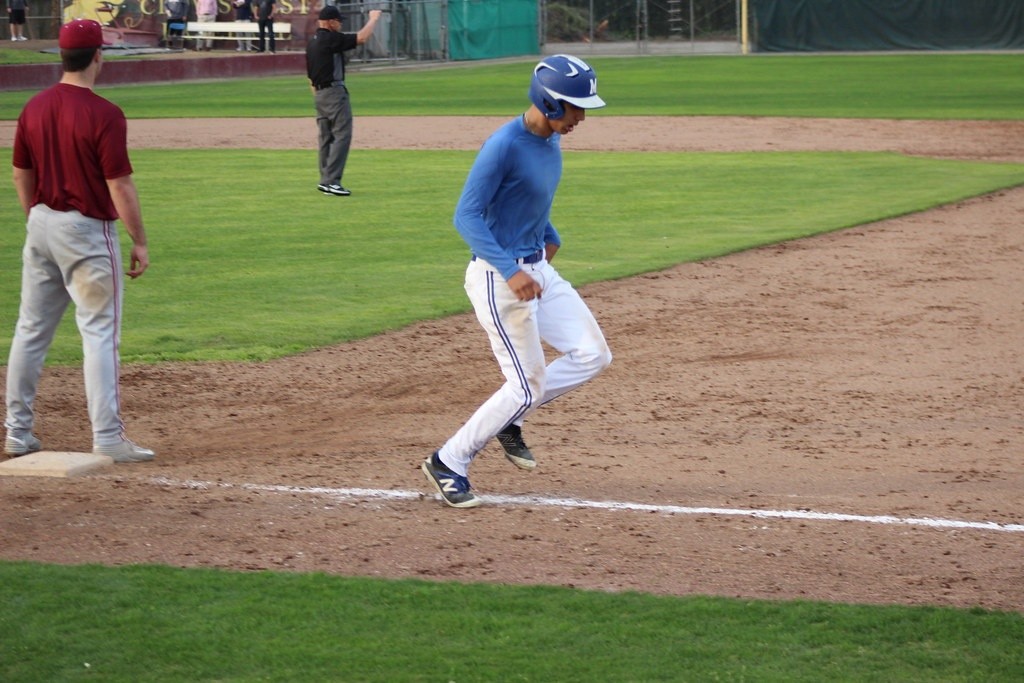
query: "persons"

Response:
[195,0,218,51]
[6,0,29,41]
[419,54,612,507]
[305,6,381,194]
[164,0,190,47]
[3,19,154,461]
[254,0,277,54]
[232,0,253,52]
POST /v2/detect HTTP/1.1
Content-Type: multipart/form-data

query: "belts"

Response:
[316,80,344,91]
[472,253,544,265]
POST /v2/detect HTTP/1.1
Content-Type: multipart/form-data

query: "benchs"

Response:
[161,20,292,49]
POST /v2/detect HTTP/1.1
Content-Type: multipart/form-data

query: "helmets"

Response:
[528,55,609,121]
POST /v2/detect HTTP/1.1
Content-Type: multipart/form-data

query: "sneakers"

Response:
[92,436,155,464]
[496,421,537,473]
[421,450,481,509]
[5,433,41,455]
[318,180,351,195]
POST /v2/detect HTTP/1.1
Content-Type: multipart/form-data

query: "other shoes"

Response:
[12,35,29,42]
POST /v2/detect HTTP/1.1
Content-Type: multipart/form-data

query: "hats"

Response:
[59,19,114,51]
[318,6,349,21]
